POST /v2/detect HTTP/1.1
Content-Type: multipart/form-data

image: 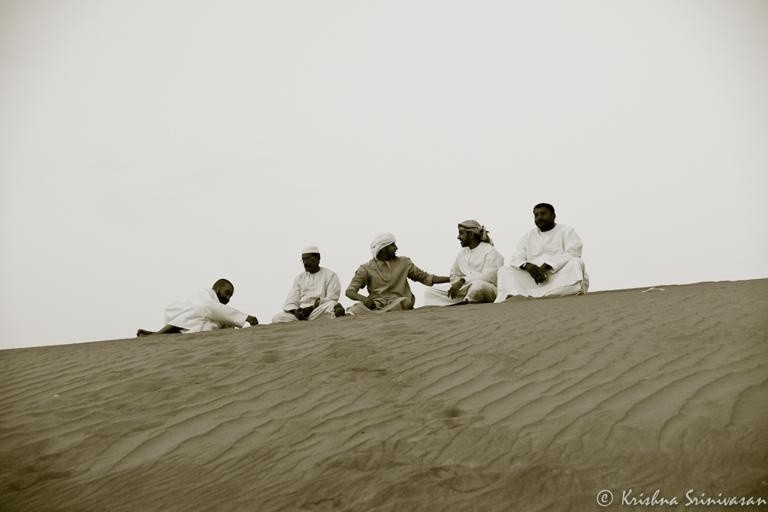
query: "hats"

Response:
[302,246,320,254]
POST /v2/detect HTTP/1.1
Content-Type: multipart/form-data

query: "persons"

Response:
[494,203,589,303]
[333,233,450,317]
[137,279,258,337]
[422,220,504,306]
[271,246,341,323]
